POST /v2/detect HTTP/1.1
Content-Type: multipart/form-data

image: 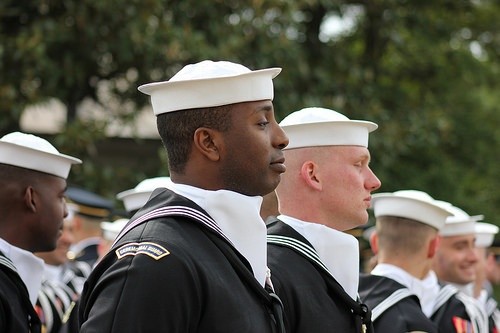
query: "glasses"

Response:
[490,254,500,263]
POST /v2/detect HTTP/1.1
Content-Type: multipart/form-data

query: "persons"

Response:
[266,107,382,333]
[0,131,82,333]
[37,60,289,333]
[359,189,500,333]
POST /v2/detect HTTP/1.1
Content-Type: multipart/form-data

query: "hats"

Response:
[117,177,174,214]
[475,222,499,248]
[371,190,455,231]
[0,133,83,178]
[66,187,115,220]
[138,60,281,117]
[100,218,130,242]
[438,205,484,236]
[279,107,378,150]
